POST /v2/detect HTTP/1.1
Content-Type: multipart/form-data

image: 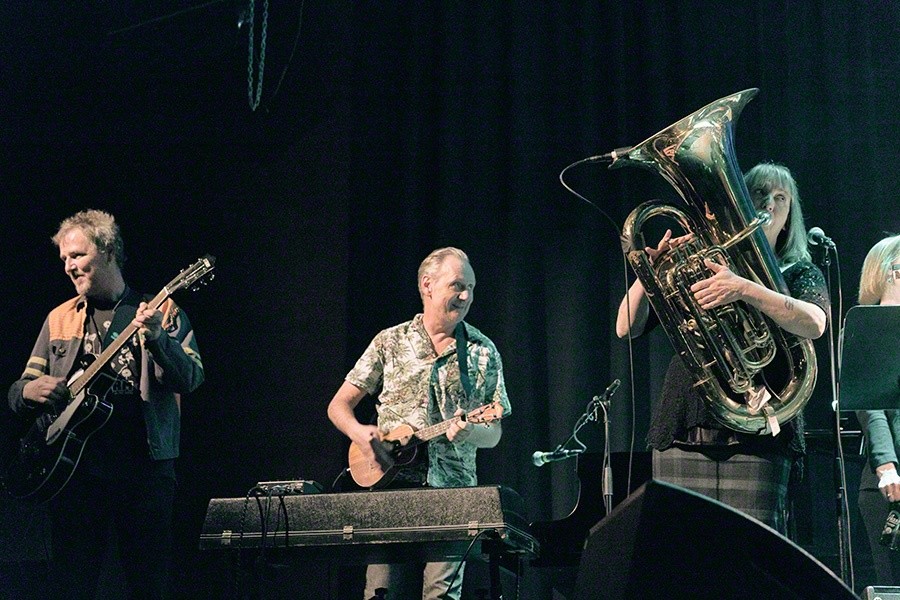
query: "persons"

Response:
[327,247,512,600]
[616,163,829,538]
[855,236,900,586]
[7,208,204,600]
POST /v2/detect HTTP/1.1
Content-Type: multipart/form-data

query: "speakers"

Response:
[859,586,900,600]
[575,477,861,600]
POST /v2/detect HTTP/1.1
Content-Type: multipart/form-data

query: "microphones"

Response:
[807,226,836,249]
[586,146,633,163]
[531,450,582,467]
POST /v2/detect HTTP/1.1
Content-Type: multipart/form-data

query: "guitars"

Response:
[0,251,217,506]
[347,401,505,489]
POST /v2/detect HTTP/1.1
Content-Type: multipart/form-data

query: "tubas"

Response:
[606,84,820,439]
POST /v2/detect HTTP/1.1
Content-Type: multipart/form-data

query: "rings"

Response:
[887,493,893,497]
